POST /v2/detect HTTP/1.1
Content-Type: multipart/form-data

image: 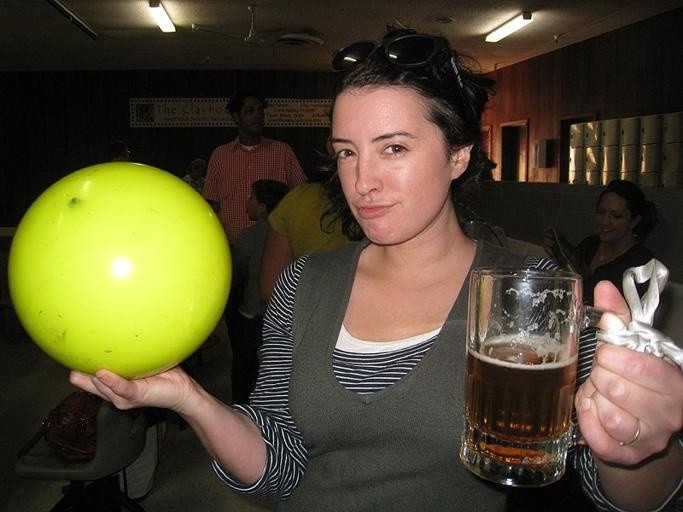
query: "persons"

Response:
[543,179,670,332]
[258,182,361,306]
[107,140,131,163]
[70,32,683,510]
[202,90,308,255]
[194,158,205,177]
[182,160,205,194]
[223,179,291,406]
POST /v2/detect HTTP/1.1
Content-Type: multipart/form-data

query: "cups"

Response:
[459,267,631,487]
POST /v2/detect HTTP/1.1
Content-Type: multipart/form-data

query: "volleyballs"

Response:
[8,161,234,379]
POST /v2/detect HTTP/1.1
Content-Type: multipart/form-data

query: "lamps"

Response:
[485,11,532,43]
[148,0,177,34]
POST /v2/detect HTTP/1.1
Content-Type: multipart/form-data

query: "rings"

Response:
[619,418,641,447]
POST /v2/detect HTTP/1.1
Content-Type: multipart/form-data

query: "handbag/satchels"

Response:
[42,390,102,463]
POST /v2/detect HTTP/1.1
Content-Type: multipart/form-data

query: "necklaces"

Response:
[598,239,633,262]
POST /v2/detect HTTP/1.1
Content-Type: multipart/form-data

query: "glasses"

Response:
[329,33,467,100]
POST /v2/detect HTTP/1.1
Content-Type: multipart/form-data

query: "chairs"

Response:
[15,401,147,512]
